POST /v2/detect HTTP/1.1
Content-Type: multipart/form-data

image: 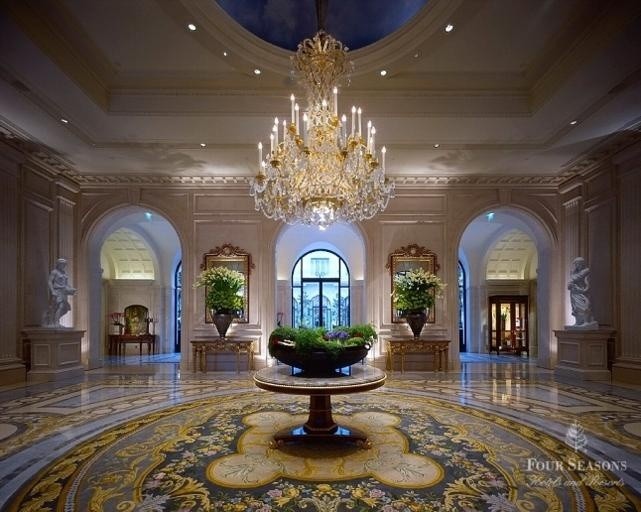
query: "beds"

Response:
[108,334,156,356]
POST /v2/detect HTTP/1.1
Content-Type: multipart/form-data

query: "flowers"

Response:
[389,266,449,311]
[191,265,247,311]
[111,312,125,324]
[267,323,378,368]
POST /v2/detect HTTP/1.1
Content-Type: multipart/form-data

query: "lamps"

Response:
[245,29,396,232]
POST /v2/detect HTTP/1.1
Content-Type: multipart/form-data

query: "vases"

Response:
[209,308,236,337]
[404,309,430,340]
[119,324,123,335]
[272,340,368,378]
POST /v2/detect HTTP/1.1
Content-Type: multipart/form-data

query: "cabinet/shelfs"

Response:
[488,294,530,357]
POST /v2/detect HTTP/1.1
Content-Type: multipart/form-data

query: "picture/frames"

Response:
[200,242,255,324]
[385,242,440,324]
